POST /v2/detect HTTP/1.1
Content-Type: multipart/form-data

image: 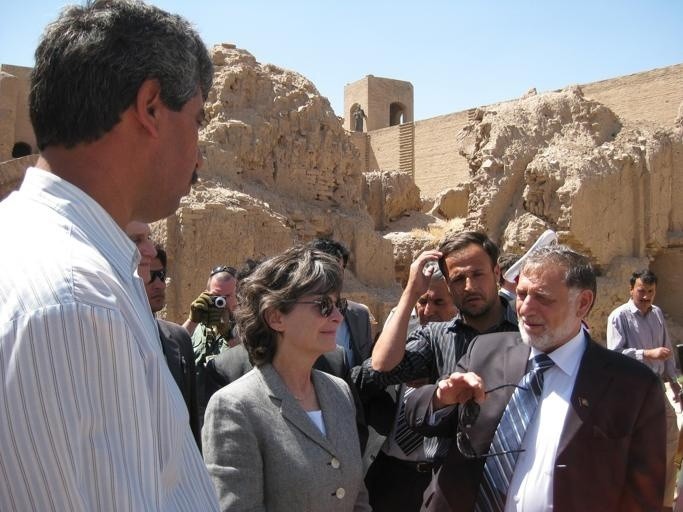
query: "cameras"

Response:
[201,296,227,312]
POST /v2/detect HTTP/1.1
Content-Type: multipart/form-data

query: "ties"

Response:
[471,353,554,511]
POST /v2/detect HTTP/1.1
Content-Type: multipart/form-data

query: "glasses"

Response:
[455,384,528,458]
[284,295,348,317]
[149,267,167,284]
[208,264,237,276]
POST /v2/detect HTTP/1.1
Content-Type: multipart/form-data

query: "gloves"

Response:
[189,290,237,339]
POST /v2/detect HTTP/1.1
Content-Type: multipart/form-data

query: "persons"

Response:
[201,241,371,457]
[201,246,374,511]
[365,231,520,512]
[405,244,667,511]
[0,1,225,511]
[351,104,367,133]
[142,239,202,460]
[181,263,242,407]
[607,268,683,511]
[123,219,157,284]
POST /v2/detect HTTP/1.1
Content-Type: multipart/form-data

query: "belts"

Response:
[374,461,436,474]
